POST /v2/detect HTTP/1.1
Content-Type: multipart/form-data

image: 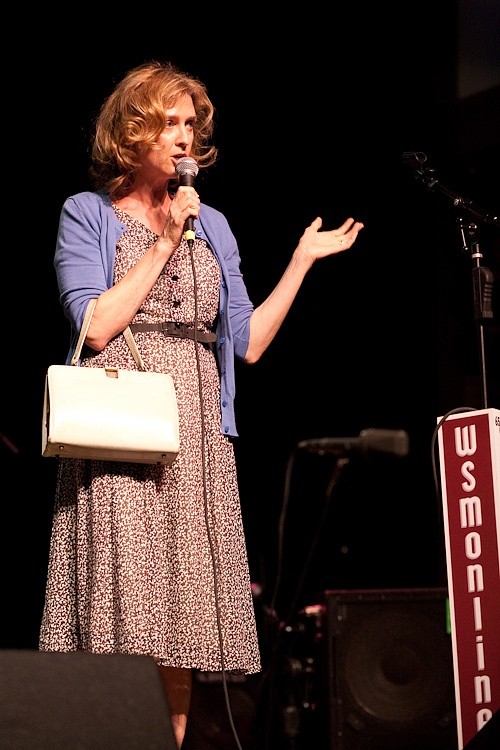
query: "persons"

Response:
[39,64,365,748]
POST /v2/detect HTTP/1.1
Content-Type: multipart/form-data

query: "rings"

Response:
[341,240,343,244]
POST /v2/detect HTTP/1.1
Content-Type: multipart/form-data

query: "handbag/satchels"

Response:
[41,299,179,465]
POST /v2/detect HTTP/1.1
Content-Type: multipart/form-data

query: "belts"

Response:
[126,320,217,344]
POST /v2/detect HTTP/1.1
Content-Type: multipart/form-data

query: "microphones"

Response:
[299,428,409,458]
[174,156,200,248]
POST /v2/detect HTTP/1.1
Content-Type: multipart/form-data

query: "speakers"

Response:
[323,587,459,750]
[0,648,178,750]
